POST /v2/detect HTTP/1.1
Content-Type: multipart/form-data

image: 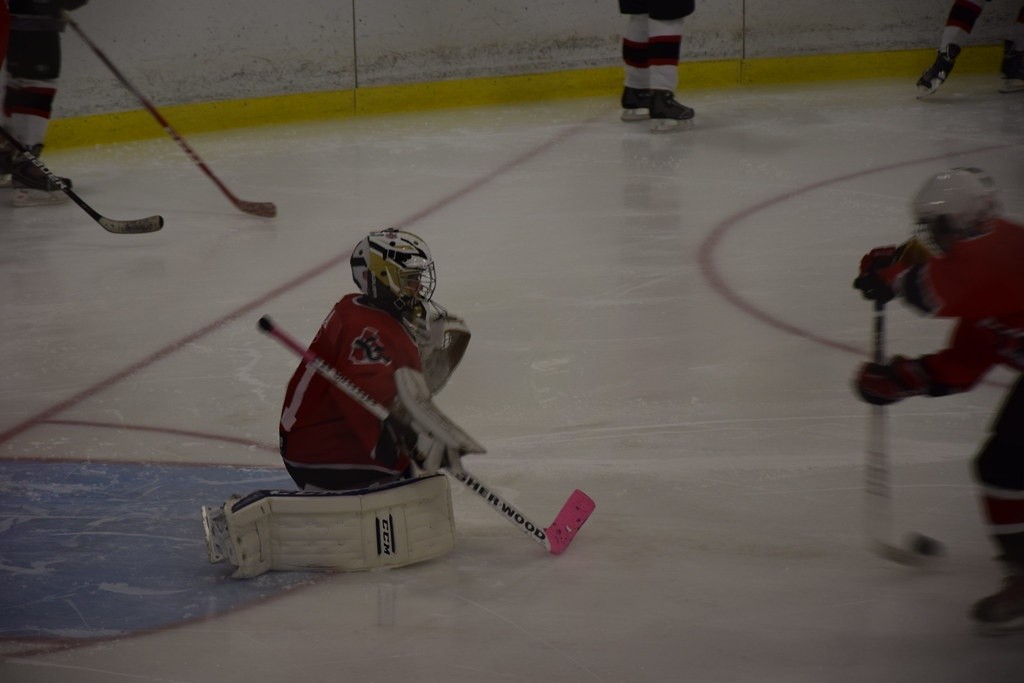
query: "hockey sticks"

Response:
[859,299,971,578]
[254,313,597,556]
[61,7,279,219]
[0,125,165,236]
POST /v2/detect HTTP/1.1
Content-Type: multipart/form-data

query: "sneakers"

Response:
[648,88,696,134]
[998,39,1024,93]
[0,130,13,187]
[915,43,961,101]
[965,553,1024,637]
[620,86,651,120]
[10,143,73,207]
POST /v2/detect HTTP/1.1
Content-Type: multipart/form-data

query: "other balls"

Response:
[911,533,941,559]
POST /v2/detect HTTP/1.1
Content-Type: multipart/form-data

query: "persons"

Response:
[852,169,1023,624]
[914,0,1024,99]
[1,0,87,206]
[279,228,471,494]
[619,1,698,132]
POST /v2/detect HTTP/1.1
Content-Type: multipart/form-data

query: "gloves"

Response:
[853,245,905,305]
[851,357,913,406]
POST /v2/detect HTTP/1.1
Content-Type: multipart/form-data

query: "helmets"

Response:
[350,228,437,310]
[908,166,1004,259]
